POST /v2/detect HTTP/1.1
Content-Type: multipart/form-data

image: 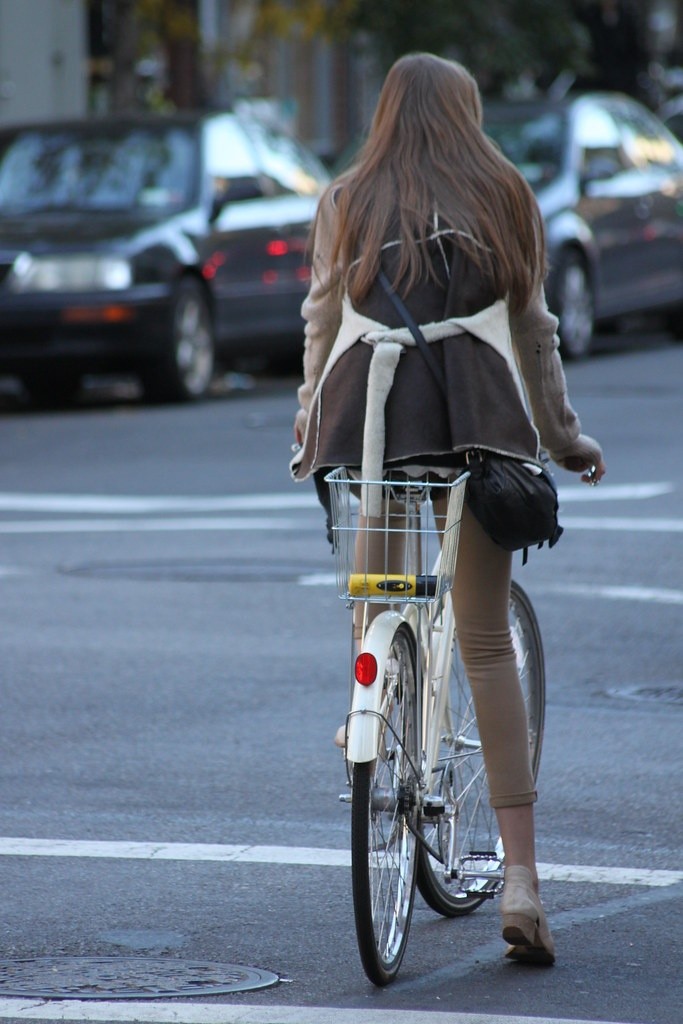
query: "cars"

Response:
[484,89,682,365]
[0,108,339,412]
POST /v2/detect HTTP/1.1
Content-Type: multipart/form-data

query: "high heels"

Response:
[335,724,380,776]
[501,865,556,965]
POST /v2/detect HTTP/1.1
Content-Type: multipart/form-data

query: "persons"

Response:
[290,50,605,965]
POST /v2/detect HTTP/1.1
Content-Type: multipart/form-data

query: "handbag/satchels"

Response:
[465,450,563,552]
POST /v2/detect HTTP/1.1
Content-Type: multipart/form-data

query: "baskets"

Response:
[324,467,471,603]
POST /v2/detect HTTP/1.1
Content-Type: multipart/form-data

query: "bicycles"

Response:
[291,424,600,987]
